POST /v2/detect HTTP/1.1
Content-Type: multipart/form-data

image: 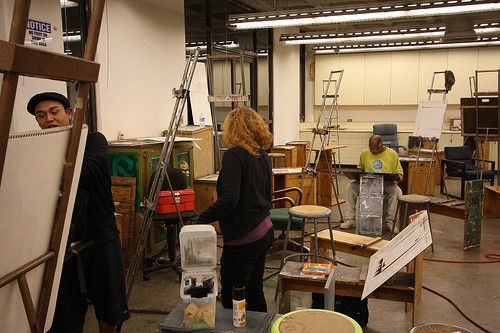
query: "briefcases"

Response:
[155,188,195,214]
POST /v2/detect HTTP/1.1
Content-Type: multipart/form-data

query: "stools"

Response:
[390,193,434,253]
[273,204,337,302]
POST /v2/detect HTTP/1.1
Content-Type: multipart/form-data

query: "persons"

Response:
[340,135,403,233]
[27,92,130,333]
[196,108,274,312]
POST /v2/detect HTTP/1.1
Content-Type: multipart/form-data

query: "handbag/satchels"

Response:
[311,292,368,329]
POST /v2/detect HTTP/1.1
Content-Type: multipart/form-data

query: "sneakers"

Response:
[385,221,399,234]
[340,219,356,229]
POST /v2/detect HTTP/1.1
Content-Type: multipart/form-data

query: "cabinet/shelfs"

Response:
[299,45,500,165]
[205,56,269,107]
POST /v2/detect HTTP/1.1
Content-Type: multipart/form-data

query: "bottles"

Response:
[232,285,246,328]
[200,112,205,128]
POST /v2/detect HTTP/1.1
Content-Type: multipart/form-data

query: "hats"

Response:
[27,92,70,116]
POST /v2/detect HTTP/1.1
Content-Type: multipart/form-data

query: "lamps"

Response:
[184,0,500,57]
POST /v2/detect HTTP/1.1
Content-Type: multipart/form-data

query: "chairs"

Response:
[439,146,495,200]
[266,185,311,260]
[142,167,199,284]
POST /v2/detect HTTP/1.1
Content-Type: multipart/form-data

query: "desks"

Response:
[105,122,448,273]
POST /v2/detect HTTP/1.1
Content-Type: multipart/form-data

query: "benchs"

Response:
[483,185,500,219]
[278,261,416,329]
[310,210,426,314]
[156,303,285,333]
